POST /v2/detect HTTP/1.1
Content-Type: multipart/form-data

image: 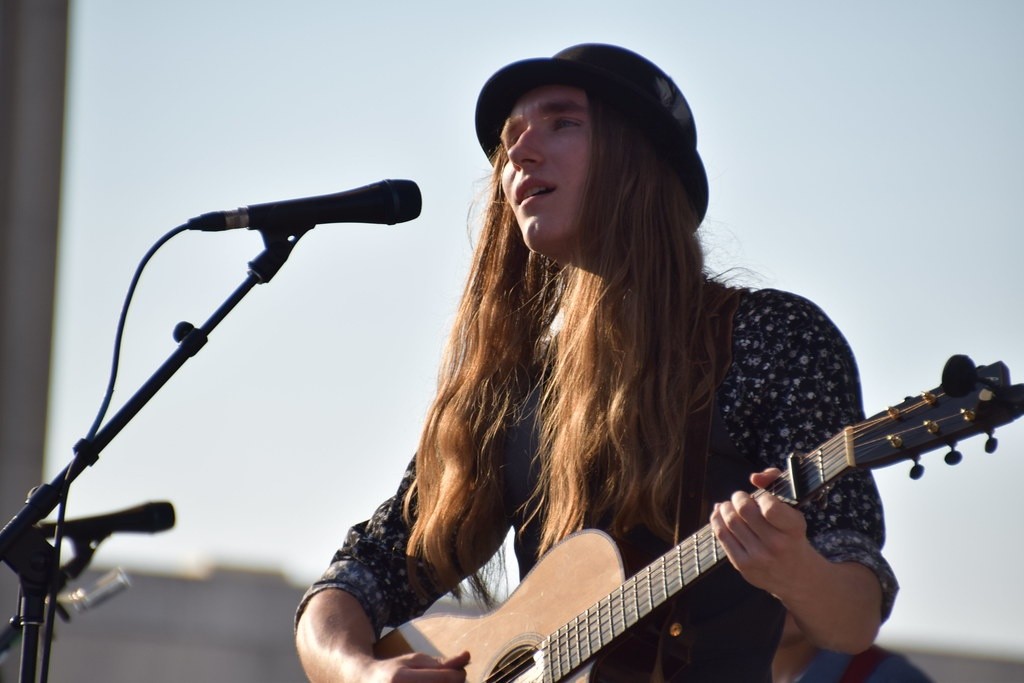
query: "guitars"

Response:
[371,355,1024,683]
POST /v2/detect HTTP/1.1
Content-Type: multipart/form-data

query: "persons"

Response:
[293,42,900,683]
[771,610,934,683]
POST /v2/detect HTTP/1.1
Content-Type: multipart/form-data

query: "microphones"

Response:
[189,178,422,232]
[38,502,176,539]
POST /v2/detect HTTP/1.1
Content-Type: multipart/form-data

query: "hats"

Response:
[475,42,709,232]
